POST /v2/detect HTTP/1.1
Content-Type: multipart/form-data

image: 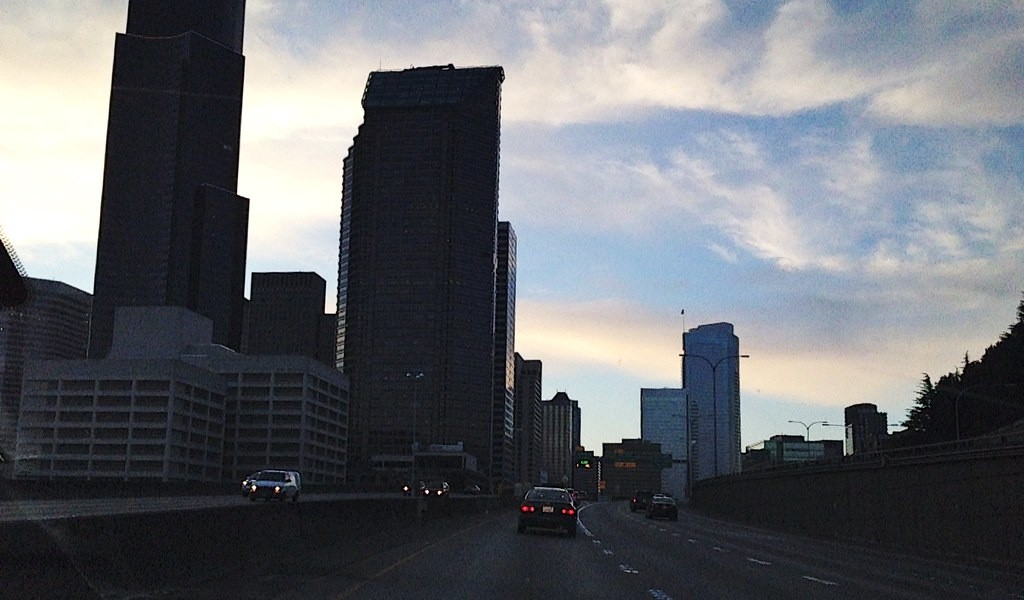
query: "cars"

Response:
[424,481,450,498]
[242,470,301,502]
[402,481,425,497]
[464,485,480,494]
[517,487,588,537]
[630,490,655,511]
[647,494,678,521]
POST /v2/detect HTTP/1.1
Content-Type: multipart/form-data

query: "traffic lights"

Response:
[576,460,593,469]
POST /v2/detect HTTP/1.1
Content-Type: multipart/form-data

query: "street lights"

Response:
[678,354,750,477]
[788,421,828,459]
[406,373,425,497]
[955,383,1018,450]
